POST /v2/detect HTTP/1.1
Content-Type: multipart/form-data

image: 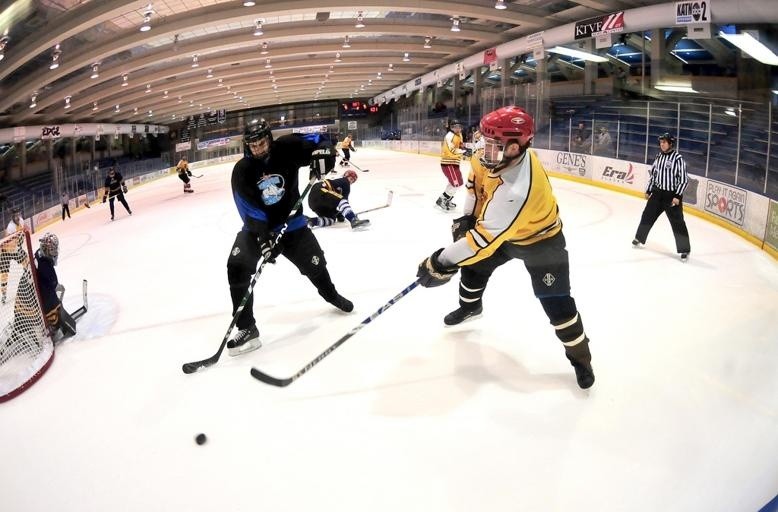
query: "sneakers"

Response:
[340,163,343,167]
[331,171,337,173]
[435,197,448,211]
[575,366,595,388]
[445,196,457,207]
[444,306,482,325]
[632,238,640,245]
[111,216,114,219]
[345,165,350,167]
[351,218,369,229]
[184,190,189,192]
[188,190,193,193]
[681,252,688,258]
[304,216,314,228]
[330,294,353,312]
[128,210,132,214]
[227,325,259,348]
[1,295,7,303]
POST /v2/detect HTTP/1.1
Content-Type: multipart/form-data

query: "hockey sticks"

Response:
[352,191,392,215]
[184,172,204,178]
[251,276,422,387]
[182,174,319,375]
[337,152,369,172]
[71,281,88,320]
[85,191,124,208]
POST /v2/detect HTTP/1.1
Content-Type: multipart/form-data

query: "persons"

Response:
[224,116,354,349]
[0,208,30,304]
[176,155,194,193]
[0,232,76,359]
[435,119,472,212]
[416,105,595,389]
[631,131,692,260]
[433,65,628,156]
[59,191,71,221]
[102,166,132,221]
[304,170,370,229]
[330,134,339,172]
[339,133,355,166]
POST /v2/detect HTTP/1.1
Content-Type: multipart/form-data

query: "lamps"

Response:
[242,0,257,7]
[495,0,508,11]
[260,42,270,56]
[89,103,255,123]
[450,15,463,31]
[89,61,101,79]
[139,4,155,32]
[423,36,436,49]
[143,68,282,107]
[253,18,267,38]
[342,36,352,48]
[316,53,412,103]
[355,11,366,28]
[265,59,273,71]
[64,96,72,109]
[0,31,11,60]
[122,72,130,86]
[191,53,200,68]
[49,44,63,70]
[29,89,41,108]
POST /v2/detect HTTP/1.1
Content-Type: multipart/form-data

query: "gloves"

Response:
[452,215,478,242]
[103,196,107,203]
[416,248,460,288]
[123,186,127,193]
[309,147,336,180]
[463,146,472,157]
[257,229,287,263]
[187,171,192,176]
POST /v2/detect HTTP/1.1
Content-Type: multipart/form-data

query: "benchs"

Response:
[0,151,170,233]
[532,94,778,202]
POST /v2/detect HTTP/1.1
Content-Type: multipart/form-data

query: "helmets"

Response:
[343,170,358,184]
[11,207,22,225]
[38,231,59,266]
[107,167,116,178]
[182,156,188,159]
[242,117,274,160]
[449,118,463,136]
[657,132,676,148]
[479,105,535,178]
